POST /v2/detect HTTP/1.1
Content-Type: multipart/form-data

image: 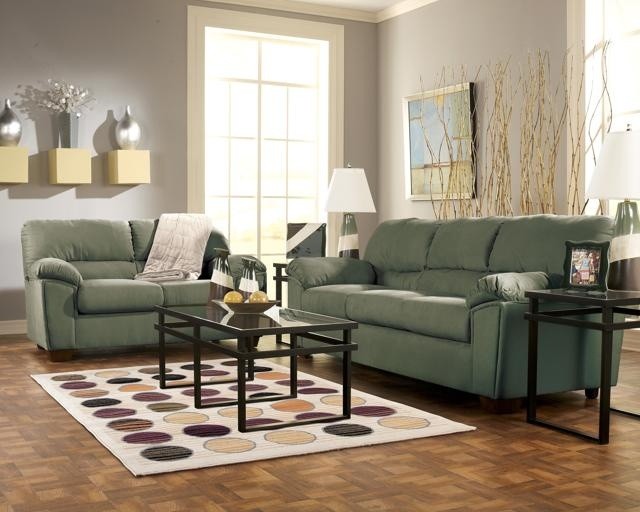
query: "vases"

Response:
[209,247,233,310]
[240,257,259,298]
[115,106,140,149]
[0,99,22,146]
[60,113,79,148]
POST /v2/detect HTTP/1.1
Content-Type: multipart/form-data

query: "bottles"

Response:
[206,306,228,322]
[207,246,261,304]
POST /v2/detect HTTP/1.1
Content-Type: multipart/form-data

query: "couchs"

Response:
[21,219,267,362]
[285,214,625,414]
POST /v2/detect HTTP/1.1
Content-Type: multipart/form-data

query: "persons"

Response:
[571,248,599,284]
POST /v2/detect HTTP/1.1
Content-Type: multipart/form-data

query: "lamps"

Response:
[324,163,376,259]
[584,124,640,291]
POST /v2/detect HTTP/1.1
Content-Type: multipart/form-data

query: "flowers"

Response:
[37,79,96,117]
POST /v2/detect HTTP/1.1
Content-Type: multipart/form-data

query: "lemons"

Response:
[249,291,268,303]
[224,291,243,303]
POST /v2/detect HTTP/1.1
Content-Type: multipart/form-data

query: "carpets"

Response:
[30,358,477,478]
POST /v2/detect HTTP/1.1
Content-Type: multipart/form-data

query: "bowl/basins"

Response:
[219,313,280,326]
[210,298,281,314]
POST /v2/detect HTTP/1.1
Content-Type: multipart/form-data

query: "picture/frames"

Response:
[403,82,477,201]
[561,240,610,292]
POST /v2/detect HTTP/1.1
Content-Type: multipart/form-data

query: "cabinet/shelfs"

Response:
[0,147,151,185]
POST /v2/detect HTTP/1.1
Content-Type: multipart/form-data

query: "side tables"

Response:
[273,263,291,346]
[523,289,640,444]
